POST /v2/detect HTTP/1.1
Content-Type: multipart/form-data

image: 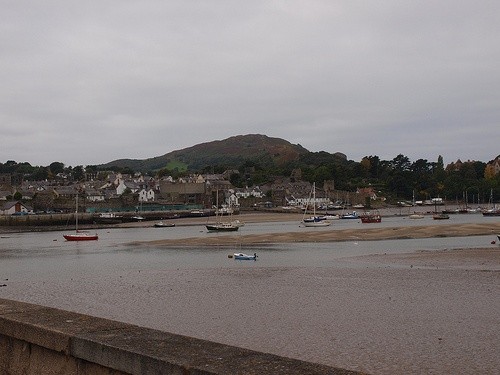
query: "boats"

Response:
[154,223,176,227]
[222,224,244,227]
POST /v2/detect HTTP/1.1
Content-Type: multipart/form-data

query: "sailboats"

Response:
[62,194,100,241]
[302,181,500,227]
[206,189,240,231]
